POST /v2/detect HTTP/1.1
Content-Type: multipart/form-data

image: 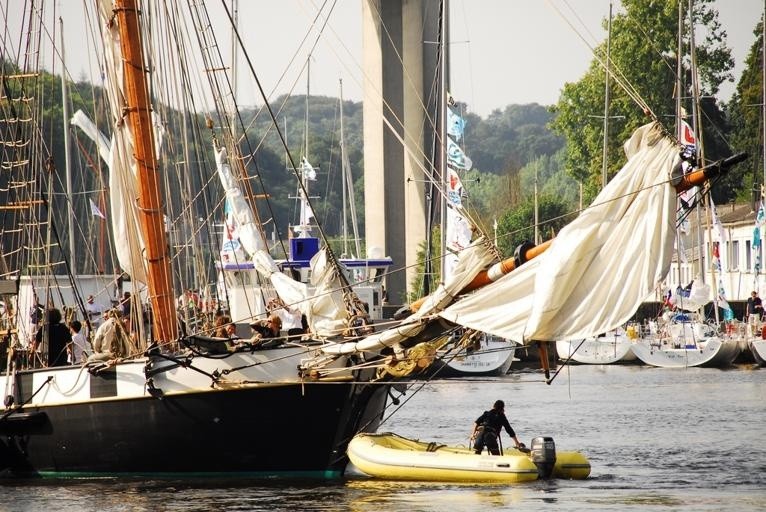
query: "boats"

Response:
[345,430,591,489]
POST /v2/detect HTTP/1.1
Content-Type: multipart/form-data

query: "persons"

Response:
[471,400,527,454]
[27,289,306,367]
[746,291,762,338]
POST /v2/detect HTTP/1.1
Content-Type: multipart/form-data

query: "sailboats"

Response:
[0,0,749,490]
[0,0,765,375]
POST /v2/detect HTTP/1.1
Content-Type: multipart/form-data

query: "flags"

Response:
[447,95,472,207]
[302,158,316,180]
[710,200,734,328]
[751,201,766,293]
[677,119,702,233]
